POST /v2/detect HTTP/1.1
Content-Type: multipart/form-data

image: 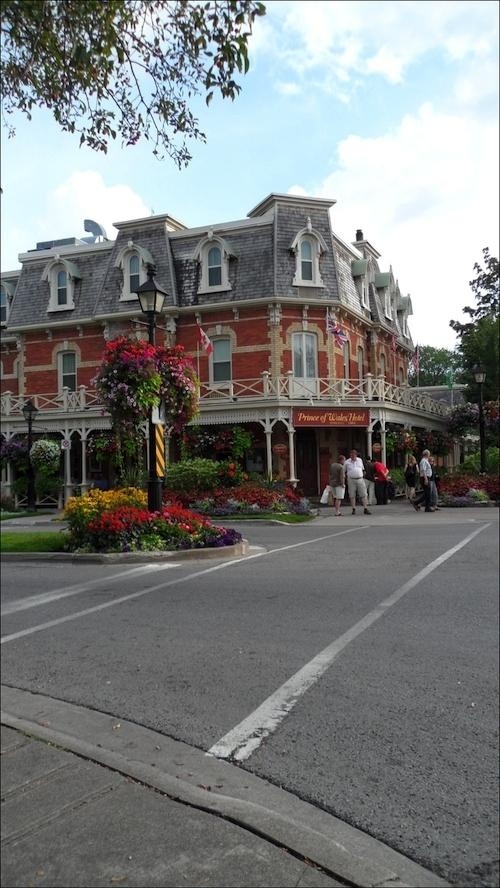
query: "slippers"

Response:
[334,512,344,516]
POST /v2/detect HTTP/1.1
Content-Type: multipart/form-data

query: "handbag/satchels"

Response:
[319,485,335,507]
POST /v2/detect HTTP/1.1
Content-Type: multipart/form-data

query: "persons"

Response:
[327,449,441,516]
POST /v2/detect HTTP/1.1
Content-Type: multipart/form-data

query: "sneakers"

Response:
[352,508,356,514]
[363,508,372,515]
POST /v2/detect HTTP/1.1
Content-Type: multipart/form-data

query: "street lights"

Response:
[471,363,490,473]
[22,400,39,514]
[131,268,172,508]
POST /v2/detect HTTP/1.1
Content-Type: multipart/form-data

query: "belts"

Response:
[349,477,362,480]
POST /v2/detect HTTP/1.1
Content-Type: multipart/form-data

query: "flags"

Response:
[325,311,348,350]
[195,317,214,356]
[411,346,419,376]
[390,333,398,353]
